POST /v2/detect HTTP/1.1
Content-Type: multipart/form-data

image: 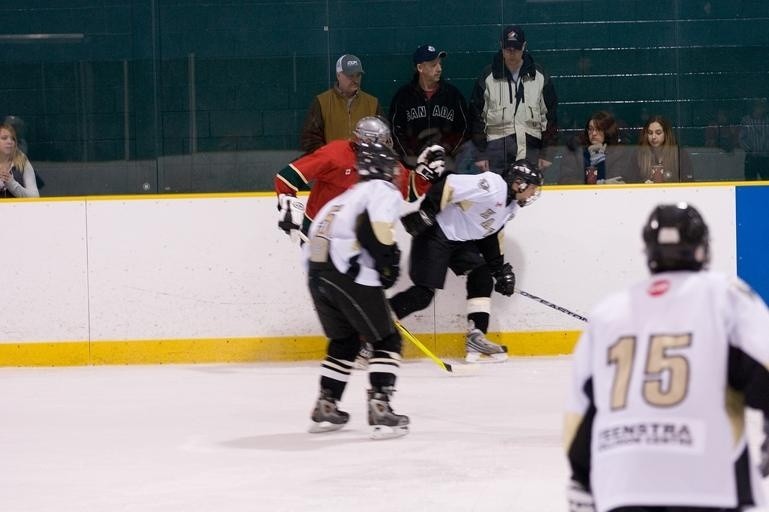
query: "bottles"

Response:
[586,162,598,185]
[652,160,665,183]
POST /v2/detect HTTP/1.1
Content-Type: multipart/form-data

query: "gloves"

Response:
[588,143,607,165]
[493,263,515,296]
[401,210,433,238]
[278,193,304,235]
[414,145,447,184]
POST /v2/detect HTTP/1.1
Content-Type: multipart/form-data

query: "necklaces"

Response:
[563,201,769,511]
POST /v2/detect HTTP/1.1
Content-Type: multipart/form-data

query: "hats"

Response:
[500,27,524,50]
[336,54,365,76]
[415,44,446,63]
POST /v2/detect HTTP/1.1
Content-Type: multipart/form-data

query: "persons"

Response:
[739,104,769,180]
[306,140,410,427]
[300,54,386,150]
[632,113,693,183]
[558,111,640,184]
[0,122,41,198]
[272,116,444,253]
[359,159,545,362]
[470,25,558,181]
[704,108,746,179]
[388,44,471,167]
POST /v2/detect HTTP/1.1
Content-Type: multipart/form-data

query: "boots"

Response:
[466,320,507,354]
[312,386,349,424]
[358,340,375,359]
[367,389,409,426]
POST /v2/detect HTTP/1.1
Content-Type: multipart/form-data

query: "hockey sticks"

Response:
[298,231,496,377]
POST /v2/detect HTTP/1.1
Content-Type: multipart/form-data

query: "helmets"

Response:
[502,160,544,208]
[644,202,709,273]
[349,117,401,189]
